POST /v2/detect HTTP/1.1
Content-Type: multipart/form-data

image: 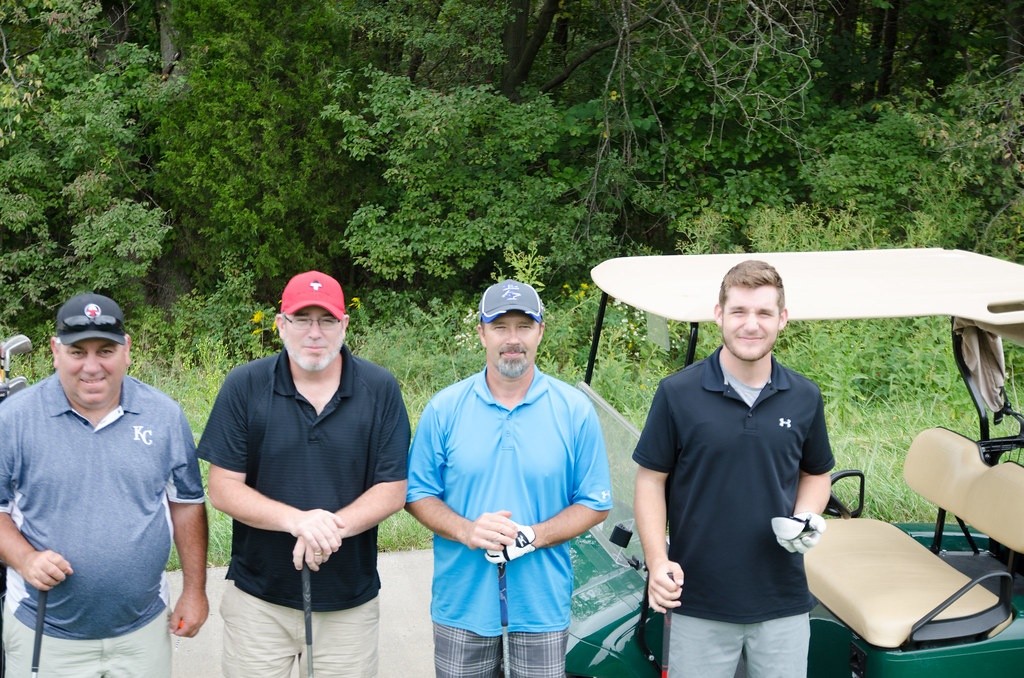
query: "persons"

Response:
[196,272,411,678]
[405,280,612,678]
[0,295,208,678]
[632,260,836,678]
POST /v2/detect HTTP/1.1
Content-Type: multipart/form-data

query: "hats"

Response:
[280,270,346,322]
[478,279,545,324]
[55,290,129,346]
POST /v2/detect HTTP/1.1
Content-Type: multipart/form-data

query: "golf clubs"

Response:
[30,589,48,678]
[300,552,315,678]
[497,562,511,678]
[661,571,675,678]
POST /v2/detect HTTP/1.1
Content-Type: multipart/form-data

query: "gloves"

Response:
[480,524,537,564]
[775,512,826,554]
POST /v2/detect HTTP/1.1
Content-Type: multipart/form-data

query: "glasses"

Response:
[284,311,340,331]
[54,316,128,336]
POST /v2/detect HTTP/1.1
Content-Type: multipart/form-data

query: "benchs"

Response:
[804,426,1024,649]
